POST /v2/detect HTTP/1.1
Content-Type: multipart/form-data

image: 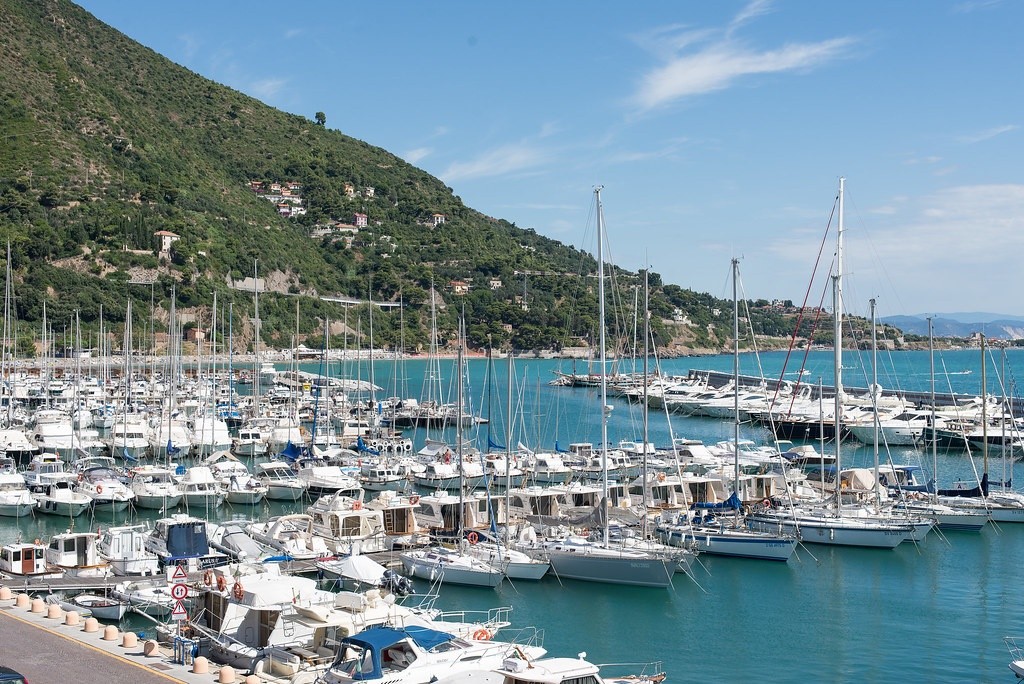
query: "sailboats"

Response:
[0,178,1024,683]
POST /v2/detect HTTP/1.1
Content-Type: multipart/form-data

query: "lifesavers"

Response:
[203,569,212,587]
[763,499,772,509]
[473,628,491,645]
[351,499,363,512]
[233,580,245,601]
[96,485,104,495]
[216,575,226,591]
[468,532,479,545]
[77,474,84,482]
[409,496,419,507]
